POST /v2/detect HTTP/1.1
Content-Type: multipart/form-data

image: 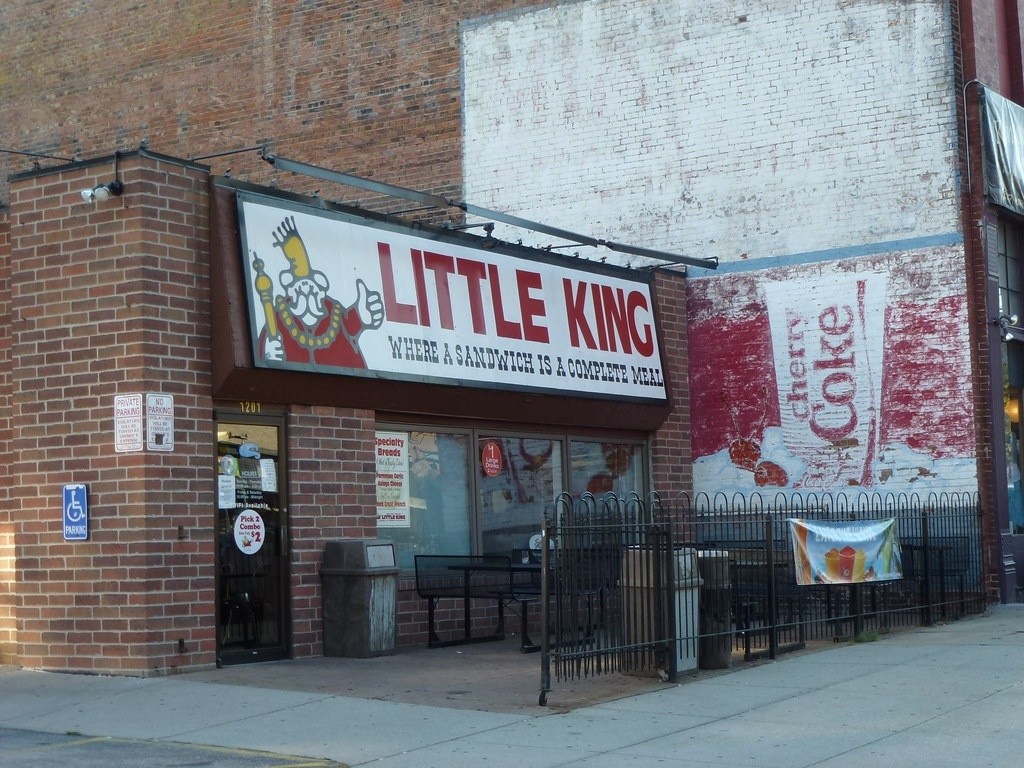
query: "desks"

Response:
[445,557,597,653]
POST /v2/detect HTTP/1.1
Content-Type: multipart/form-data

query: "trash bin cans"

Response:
[616,545,704,679]
[318,540,399,660]
[696,549,733,669]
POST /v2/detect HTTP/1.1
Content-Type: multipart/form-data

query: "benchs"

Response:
[413,554,543,654]
[676,535,971,657]
[493,546,620,645]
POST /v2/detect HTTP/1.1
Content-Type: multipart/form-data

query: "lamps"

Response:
[81,179,122,204]
[993,314,1019,342]
